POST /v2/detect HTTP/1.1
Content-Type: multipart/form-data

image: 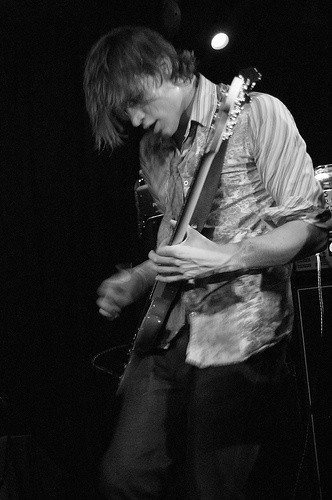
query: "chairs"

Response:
[0,398,69,500]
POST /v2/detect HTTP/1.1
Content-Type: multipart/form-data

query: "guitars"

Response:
[114,64,263,394]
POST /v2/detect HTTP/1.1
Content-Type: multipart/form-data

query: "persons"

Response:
[85,25,332,500]
